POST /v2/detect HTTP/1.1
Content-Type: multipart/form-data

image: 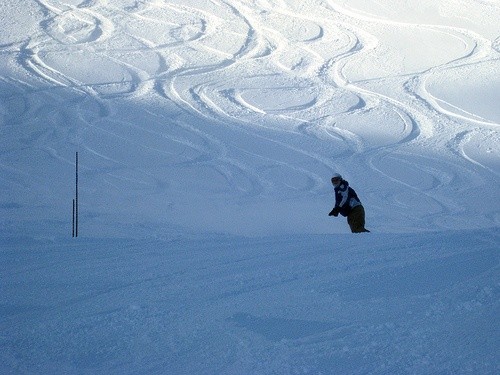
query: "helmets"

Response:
[330,172,341,179]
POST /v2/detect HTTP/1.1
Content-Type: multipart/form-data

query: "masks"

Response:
[331,181,341,187]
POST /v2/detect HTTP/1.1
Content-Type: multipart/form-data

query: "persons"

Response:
[328,172,371,234]
